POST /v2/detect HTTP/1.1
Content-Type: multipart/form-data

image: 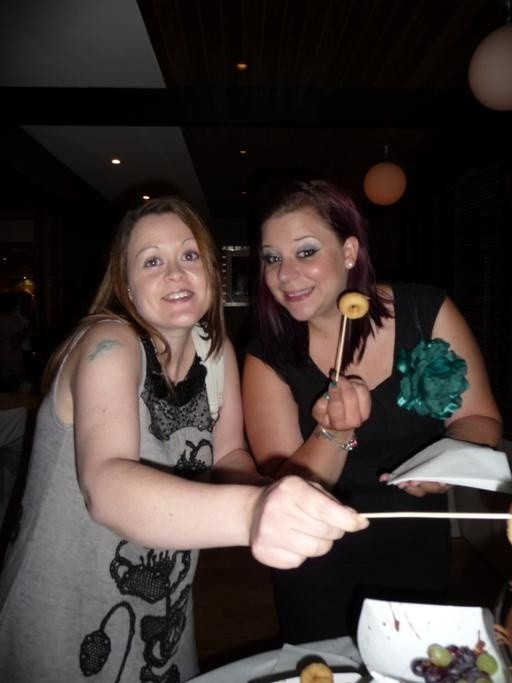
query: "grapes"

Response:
[412,644,498,683]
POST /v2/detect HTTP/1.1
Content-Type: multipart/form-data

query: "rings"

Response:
[329,394,344,403]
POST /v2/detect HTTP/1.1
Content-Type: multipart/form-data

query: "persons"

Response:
[2,196,371,683]
[245,172,506,648]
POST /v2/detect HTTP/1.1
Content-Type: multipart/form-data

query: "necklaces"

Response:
[149,331,185,410]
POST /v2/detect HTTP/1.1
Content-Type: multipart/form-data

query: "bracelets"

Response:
[312,418,362,450]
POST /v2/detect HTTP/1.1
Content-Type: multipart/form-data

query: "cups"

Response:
[356,595,509,683]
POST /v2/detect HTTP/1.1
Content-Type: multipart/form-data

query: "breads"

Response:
[300,662,333,683]
